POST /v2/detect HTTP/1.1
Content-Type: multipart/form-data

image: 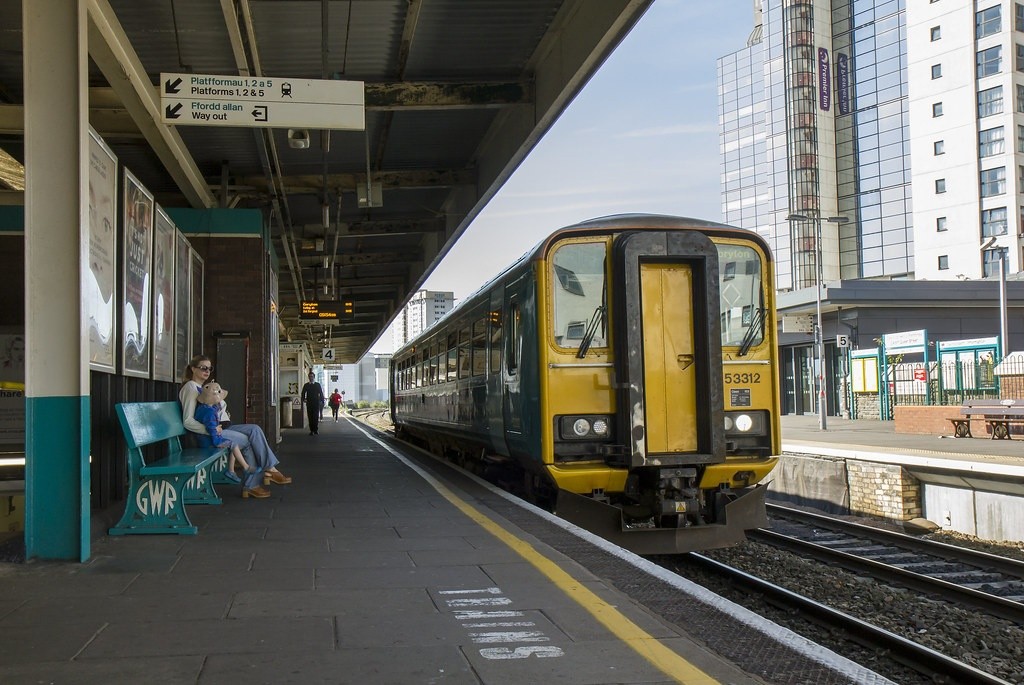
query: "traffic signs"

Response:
[161,72,365,131]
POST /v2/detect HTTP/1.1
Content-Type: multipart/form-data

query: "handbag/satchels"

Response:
[328,401,332,406]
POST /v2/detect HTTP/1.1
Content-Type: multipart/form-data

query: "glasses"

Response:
[194,365,213,373]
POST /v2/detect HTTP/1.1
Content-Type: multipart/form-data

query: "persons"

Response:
[328,388,344,423]
[178,355,292,497]
[302,372,324,436]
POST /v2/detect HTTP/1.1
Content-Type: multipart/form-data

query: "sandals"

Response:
[242,466,262,475]
[224,469,241,483]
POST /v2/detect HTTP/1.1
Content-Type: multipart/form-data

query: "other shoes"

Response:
[314,432,318,435]
[309,433,313,435]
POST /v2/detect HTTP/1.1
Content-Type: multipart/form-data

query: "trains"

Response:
[389,212,782,554]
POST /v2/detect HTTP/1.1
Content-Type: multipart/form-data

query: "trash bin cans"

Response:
[280,397,293,428]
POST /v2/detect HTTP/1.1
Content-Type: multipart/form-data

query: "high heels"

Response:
[242,485,271,498]
[263,470,292,485]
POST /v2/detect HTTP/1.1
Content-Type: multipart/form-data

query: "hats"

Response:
[196,379,228,406]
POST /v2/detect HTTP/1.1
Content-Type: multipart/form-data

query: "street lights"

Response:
[784,214,851,430]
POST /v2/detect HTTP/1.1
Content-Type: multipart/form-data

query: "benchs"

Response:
[109,402,238,535]
[946,399,1024,440]
